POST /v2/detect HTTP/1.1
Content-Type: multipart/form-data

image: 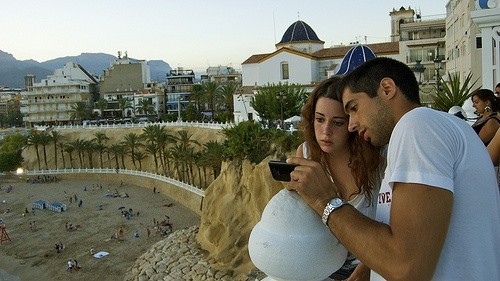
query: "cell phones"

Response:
[268,160,300,182]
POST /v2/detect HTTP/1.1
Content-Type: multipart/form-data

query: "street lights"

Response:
[276,89,287,129]
[433,55,441,97]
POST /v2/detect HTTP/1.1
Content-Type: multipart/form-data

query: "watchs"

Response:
[321,197,349,228]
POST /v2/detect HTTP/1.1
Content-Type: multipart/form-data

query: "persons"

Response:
[296,76,389,280]
[285,57,500,281]
[471,82,500,172]
[0,174,175,274]
